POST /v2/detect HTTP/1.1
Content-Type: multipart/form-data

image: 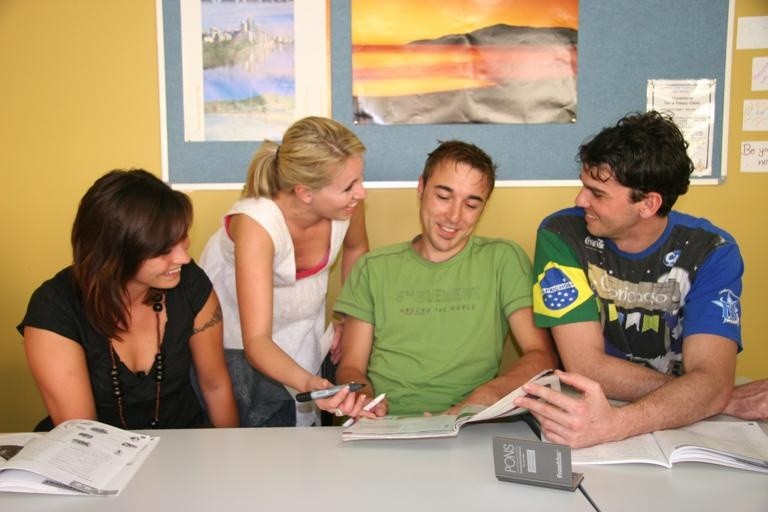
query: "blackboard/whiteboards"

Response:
[157,2,736,191]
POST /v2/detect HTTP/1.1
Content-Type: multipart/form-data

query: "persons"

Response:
[16,170,240,433]
[189,116,371,426]
[333,140,558,418]
[513,110,767,450]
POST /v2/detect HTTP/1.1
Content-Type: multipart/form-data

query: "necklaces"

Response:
[108,289,162,429]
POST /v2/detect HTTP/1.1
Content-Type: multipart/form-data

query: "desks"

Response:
[0,418,597,512]
[531,409,768,511]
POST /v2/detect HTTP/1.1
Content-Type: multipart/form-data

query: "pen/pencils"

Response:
[340,392,387,428]
[295,384,368,403]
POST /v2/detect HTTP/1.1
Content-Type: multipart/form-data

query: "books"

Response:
[342,367,559,441]
[0,418,161,496]
[541,422,768,470]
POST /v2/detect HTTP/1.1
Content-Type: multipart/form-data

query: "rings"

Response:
[336,409,342,416]
